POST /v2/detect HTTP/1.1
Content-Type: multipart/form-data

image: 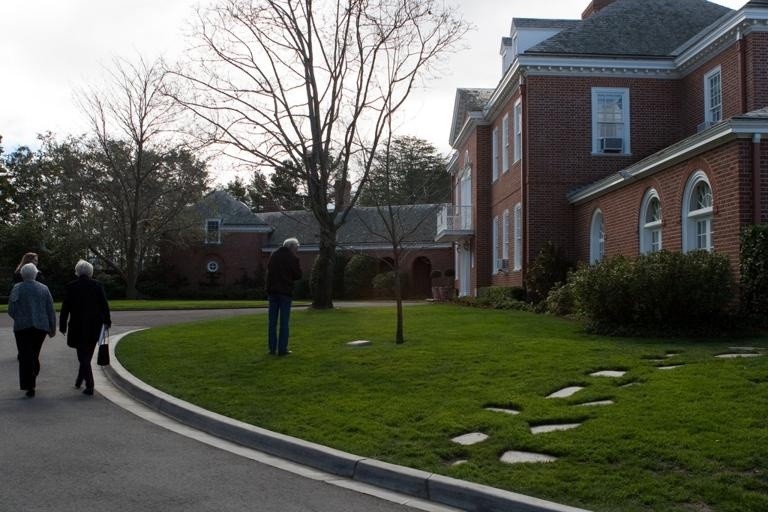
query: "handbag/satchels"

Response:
[97,326,109,366]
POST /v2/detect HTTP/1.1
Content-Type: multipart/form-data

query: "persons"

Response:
[7,262,57,399]
[264,236,305,356]
[59,257,112,396]
[14,252,47,361]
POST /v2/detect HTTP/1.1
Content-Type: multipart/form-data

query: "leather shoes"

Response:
[269,351,276,355]
[83,390,93,395]
[278,350,292,356]
